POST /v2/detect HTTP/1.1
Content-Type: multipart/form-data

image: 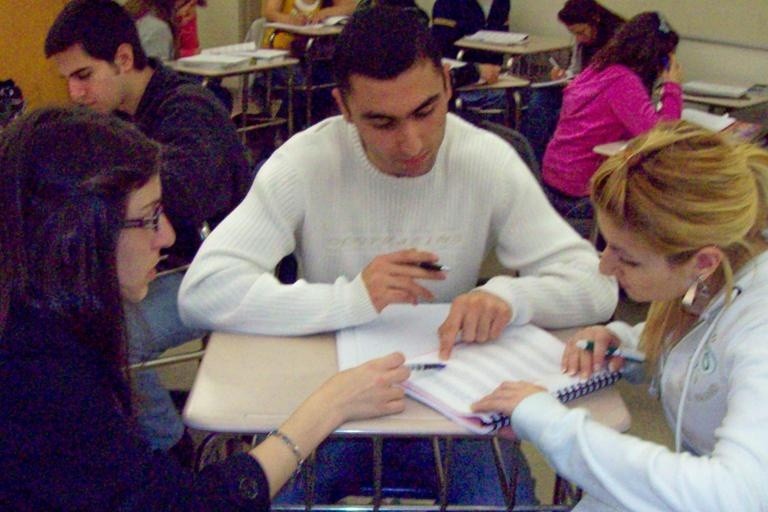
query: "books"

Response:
[389,321,622,434]
[467,30,530,45]
[176,52,248,71]
[266,15,350,31]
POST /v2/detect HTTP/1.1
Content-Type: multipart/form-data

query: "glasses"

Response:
[123,206,164,230]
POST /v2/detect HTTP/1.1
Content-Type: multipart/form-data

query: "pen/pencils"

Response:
[576,340,646,362]
[548,57,560,70]
[416,262,450,271]
[405,364,446,369]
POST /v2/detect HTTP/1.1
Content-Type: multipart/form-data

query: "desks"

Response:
[454,33,576,73]
[179,327,630,512]
[454,74,531,132]
[594,110,737,158]
[681,80,767,109]
[162,53,301,168]
[262,21,344,129]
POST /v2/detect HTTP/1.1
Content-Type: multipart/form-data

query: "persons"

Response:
[122,1,234,112]
[1,103,410,510]
[175,2,620,508]
[251,2,358,139]
[44,1,298,480]
[541,10,682,298]
[428,2,516,130]
[517,3,628,166]
[473,121,768,511]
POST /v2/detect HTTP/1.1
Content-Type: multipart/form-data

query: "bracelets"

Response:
[268,429,303,477]
